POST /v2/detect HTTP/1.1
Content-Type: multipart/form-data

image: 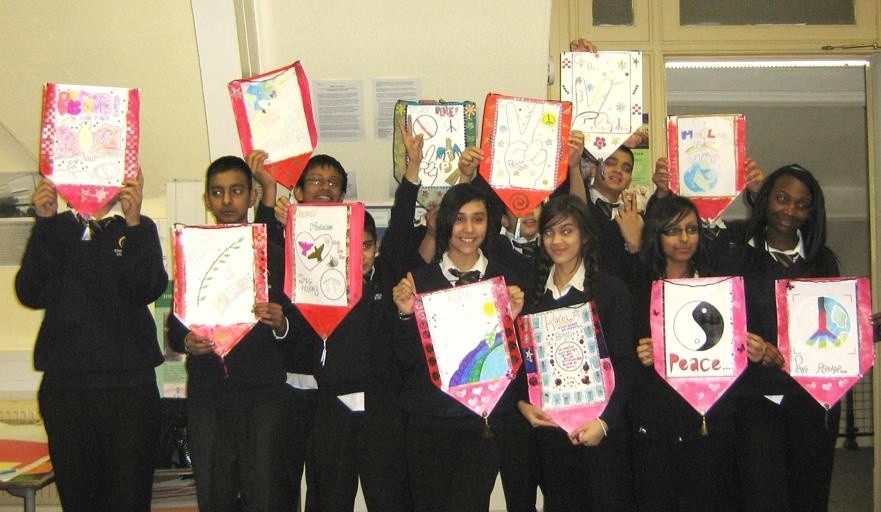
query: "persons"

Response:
[568,37,635,215]
[165,115,879,511]
[14,159,169,510]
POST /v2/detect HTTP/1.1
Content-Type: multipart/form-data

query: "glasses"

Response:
[659,223,701,238]
[302,173,344,192]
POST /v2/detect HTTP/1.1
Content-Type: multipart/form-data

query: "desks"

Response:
[0,461,56,512]
